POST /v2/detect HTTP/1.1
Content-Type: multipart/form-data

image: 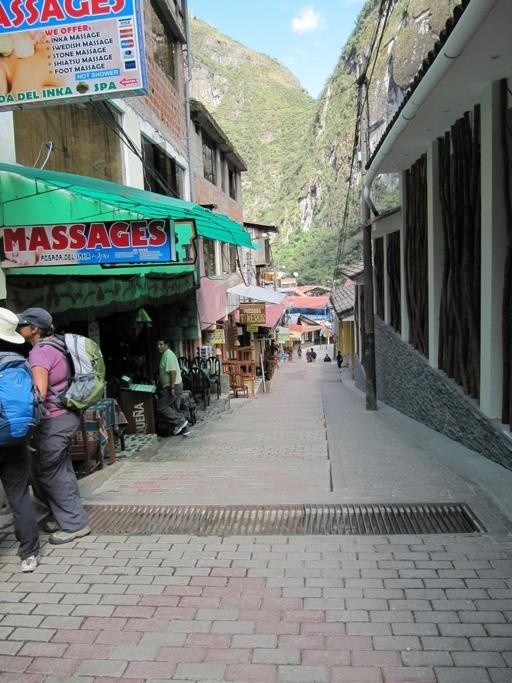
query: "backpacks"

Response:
[36,332,108,411]
[1,351,44,446]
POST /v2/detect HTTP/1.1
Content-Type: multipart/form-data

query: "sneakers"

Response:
[44,521,91,543]
[172,420,191,436]
[21,553,38,573]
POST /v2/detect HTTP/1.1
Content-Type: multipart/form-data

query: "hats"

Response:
[16,307,52,330]
[0,307,26,345]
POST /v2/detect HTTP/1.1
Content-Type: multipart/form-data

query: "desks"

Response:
[76,398,116,465]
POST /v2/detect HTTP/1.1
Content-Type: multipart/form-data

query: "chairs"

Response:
[178,356,256,409]
[114,424,126,450]
[67,416,104,478]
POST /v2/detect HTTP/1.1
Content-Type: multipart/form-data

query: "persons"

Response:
[0,306,42,575]
[17,307,93,546]
[153,338,193,438]
[295,346,344,369]
